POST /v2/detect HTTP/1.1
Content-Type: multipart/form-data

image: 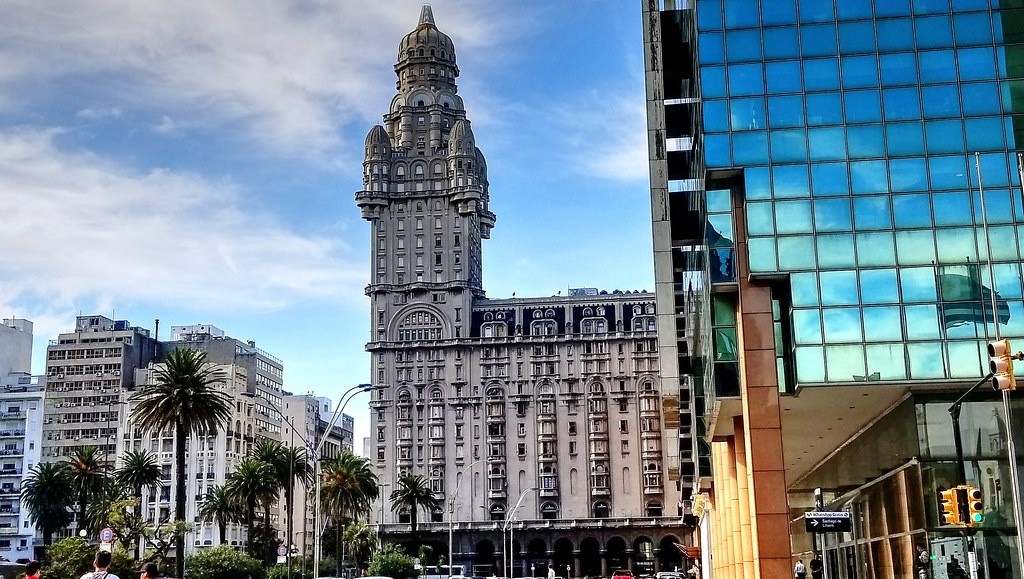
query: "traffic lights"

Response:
[938,484,985,528]
[987,339,1016,391]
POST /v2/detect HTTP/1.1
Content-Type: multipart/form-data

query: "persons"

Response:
[809,553,824,579]
[548,564,555,579]
[133,562,159,579]
[914,545,930,579]
[794,559,807,579]
[24,561,40,579]
[79,550,120,579]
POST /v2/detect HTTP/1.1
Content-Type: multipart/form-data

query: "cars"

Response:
[611,570,635,579]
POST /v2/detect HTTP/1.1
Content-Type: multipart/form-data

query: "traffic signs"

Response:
[805,511,851,532]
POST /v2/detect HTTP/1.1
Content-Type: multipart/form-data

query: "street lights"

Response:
[92,401,129,530]
[373,482,391,555]
[502,487,542,578]
[448,456,507,577]
[240,383,391,579]
[505,504,526,578]
